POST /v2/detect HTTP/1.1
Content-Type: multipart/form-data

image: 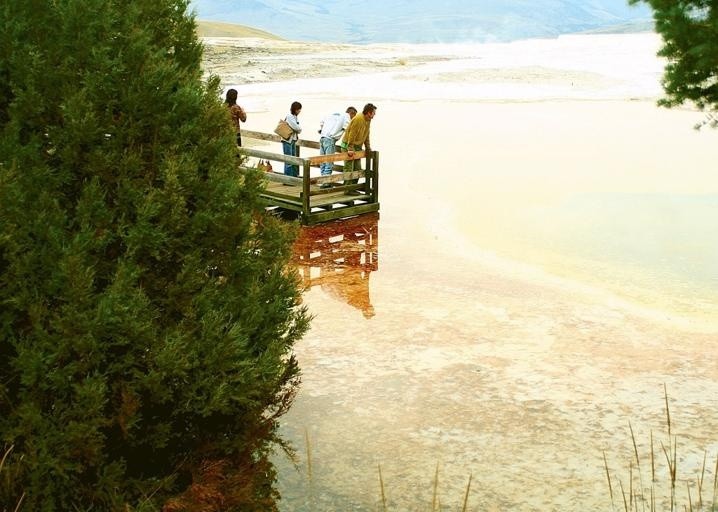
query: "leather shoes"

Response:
[319,184,330,189]
[343,189,360,196]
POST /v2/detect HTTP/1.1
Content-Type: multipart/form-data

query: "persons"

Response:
[223,89,247,159]
[281,102,303,186]
[340,103,378,195]
[319,107,357,188]
[319,267,376,319]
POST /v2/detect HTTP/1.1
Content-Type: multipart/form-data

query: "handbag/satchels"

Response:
[275,119,293,140]
